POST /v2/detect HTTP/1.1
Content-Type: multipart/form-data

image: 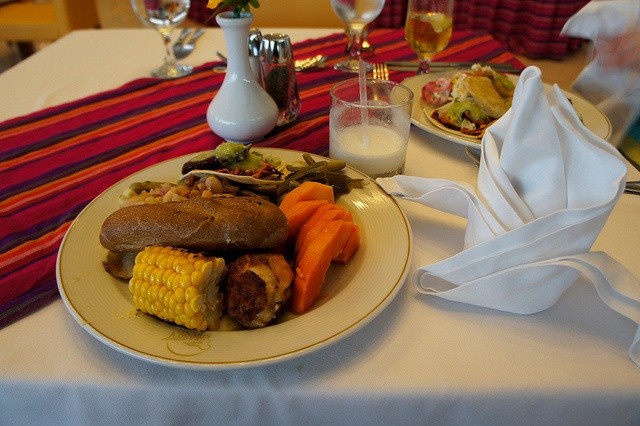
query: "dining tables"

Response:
[1,27,640,425]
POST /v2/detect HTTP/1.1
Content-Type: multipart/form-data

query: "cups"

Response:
[329,78,413,182]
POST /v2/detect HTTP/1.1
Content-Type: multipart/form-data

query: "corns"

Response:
[128,245,227,331]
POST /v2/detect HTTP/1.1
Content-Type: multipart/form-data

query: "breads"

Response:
[99,195,289,279]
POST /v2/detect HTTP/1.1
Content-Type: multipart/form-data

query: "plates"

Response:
[56,149,413,368]
[390,69,612,151]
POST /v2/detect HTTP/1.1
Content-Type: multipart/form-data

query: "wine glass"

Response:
[129,0,194,80]
[405,0,453,74]
[331,0,385,73]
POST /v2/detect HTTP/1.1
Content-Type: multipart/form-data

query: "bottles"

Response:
[249,29,262,82]
[259,28,299,126]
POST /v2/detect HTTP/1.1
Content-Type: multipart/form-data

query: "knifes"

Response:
[172,28,205,60]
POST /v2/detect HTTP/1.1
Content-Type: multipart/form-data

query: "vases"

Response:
[206,11,279,144]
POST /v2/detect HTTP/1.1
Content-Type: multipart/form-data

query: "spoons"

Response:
[212,55,328,73]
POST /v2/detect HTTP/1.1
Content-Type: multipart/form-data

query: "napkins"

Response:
[377,67,640,368]
